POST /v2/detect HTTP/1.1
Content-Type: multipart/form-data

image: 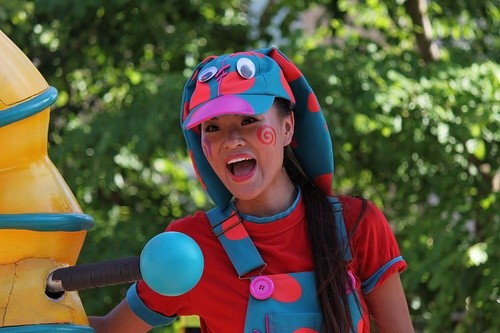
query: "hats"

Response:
[180,47,333,211]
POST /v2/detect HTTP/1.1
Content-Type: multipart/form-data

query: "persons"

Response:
[86,47,415,333]
